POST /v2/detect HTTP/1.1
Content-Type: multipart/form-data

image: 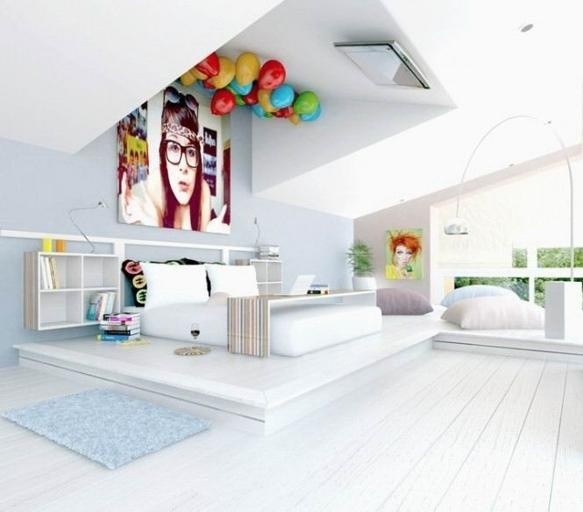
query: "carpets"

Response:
[2,389,213,469]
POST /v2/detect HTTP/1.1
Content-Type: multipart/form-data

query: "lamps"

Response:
[253,216,271,259]
[68,200,111,251]
[333,41,432,92]
[445,113,575,281]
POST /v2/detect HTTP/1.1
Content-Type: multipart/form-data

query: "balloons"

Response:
[181,52,320,127]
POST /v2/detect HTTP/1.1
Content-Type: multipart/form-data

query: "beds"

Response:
[125,259,383,355]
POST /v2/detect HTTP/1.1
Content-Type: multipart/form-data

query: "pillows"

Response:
[138,261,209,307]
[440,284,519,308]
[376,289,434,315]
[441,302,546,330]
[205,264,259,297]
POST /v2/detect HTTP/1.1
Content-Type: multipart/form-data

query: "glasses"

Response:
[164,139,202,168]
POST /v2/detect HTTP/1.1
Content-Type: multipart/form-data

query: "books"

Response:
[43,257,53,290]
[85,292,141,341]
[49,257,60,289]
[39,256,47,290]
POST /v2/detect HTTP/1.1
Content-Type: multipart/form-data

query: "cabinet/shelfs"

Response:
[22,252,121,332]
[249,258,283,296]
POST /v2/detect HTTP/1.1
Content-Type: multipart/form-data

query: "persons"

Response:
[387,235,422,280]
[116,92,231,235]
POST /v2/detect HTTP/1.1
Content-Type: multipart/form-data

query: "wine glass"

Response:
[190,323,201,346]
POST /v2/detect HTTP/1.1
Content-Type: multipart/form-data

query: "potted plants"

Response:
[347,241,377,291]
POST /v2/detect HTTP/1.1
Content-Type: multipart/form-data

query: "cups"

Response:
[42,239,53,252]
[56,239,65,253]
